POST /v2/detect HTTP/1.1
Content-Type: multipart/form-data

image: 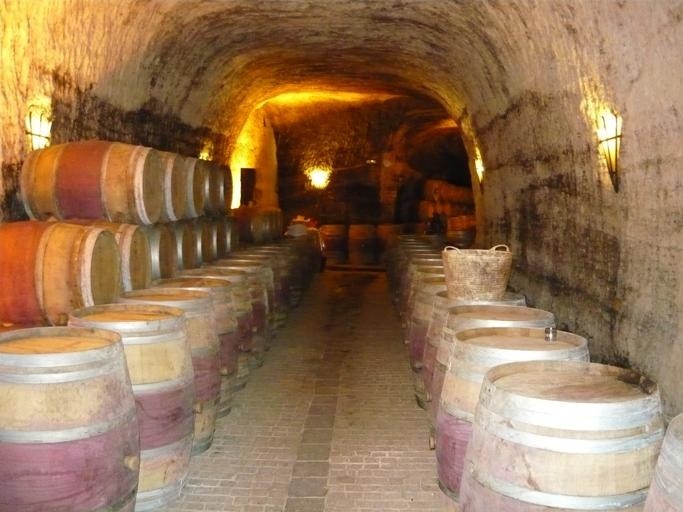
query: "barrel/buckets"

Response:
[241,203,290,242]
[318,219,398,265]
[0,219,241,301]
[18,138,239,216]
[389,229,650,510]
[0,246,307,510]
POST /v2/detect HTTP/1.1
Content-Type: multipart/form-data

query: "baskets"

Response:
[440,244,514,301]
